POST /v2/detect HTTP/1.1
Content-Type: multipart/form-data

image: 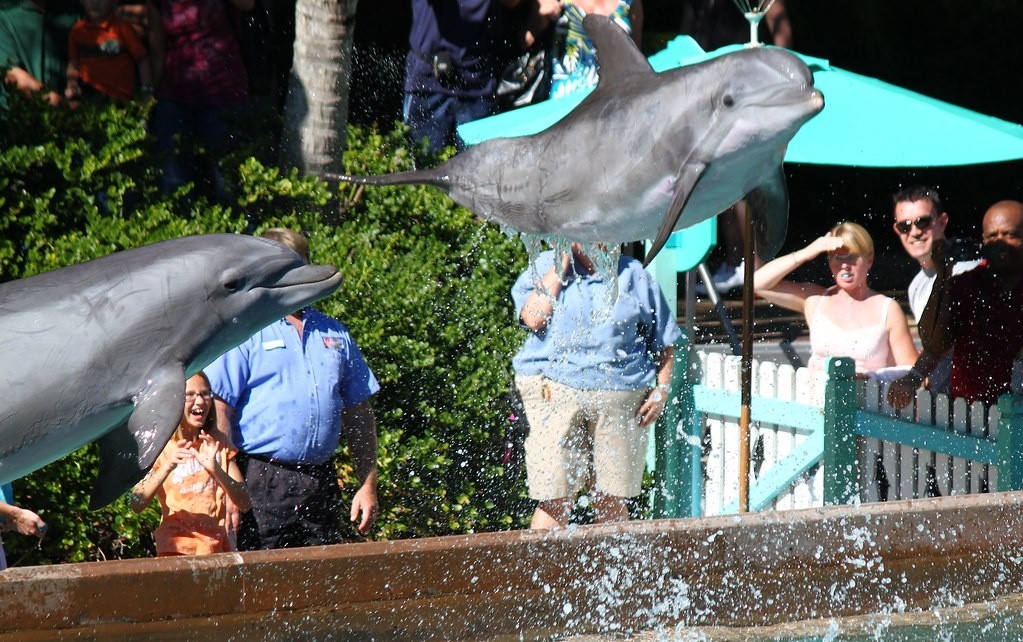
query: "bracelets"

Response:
[911,369,923,378]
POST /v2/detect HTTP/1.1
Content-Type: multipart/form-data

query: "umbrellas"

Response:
[455,42,1023,514]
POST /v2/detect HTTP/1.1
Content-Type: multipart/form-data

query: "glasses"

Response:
[827,253,859,262]
[185,390,214,400]
[895,214,938,233]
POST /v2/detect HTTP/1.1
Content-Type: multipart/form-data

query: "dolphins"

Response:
[1,233,346,513]
[318,14,823,270]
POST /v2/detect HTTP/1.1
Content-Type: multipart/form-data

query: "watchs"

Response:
[658,384,672,394]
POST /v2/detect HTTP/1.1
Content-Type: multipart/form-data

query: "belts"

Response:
[257,456,332,475]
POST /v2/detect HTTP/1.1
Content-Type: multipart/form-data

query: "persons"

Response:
[0,0,254,165]
[511,241,683,530]
[754,187,1023,410]
[402,0,643,153]
[203,228,381,550]
[676,0,794,295]
[130,371,252,557]
[0,482,46,568]
